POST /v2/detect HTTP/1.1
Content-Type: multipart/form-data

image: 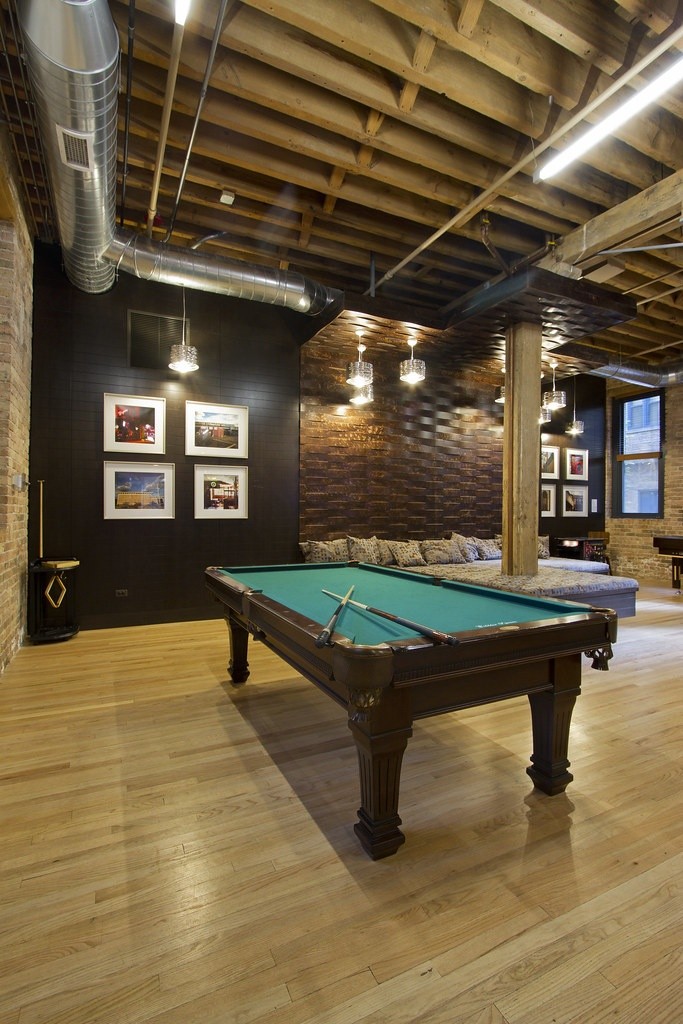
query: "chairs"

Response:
[538,557,610,575]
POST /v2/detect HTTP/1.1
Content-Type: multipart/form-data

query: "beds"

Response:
[388,558,640,619]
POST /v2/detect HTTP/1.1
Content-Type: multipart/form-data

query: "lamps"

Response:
[494,367,505,404]
[345,328,374,387]
[564,375,584,435]
[540,371,552,423]
[348,344,375,404]
[168,286,200,373]
[529,54,683,185]
[399,336,426,384]
[541,360,566,410]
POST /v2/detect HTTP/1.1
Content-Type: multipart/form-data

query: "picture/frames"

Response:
[541,445,560,480]
[183,399,249,460]
[564,447,589,481]
[194,464,249,519]
[562,484,589,517]
[541,484,556,517]
[103,392,167,455]
[103,460,175,520]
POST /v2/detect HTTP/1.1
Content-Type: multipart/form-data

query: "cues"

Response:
[314,585,355,649]
[322,589,460,647]
[38,479,45,559]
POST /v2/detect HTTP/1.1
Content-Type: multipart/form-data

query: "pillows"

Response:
[451,531,466,542]
[471,536,502,560]
[307,538,350,563]
[386,541,428,568]
[376,540,396,566]
[346,534,381,563]
[538,542,550,559]
[298,542,311,564]
[458,538,479,562]
[421,540,467,565]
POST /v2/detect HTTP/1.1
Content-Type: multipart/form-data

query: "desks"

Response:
[653,536,683,558]
[203,559,620,863]
